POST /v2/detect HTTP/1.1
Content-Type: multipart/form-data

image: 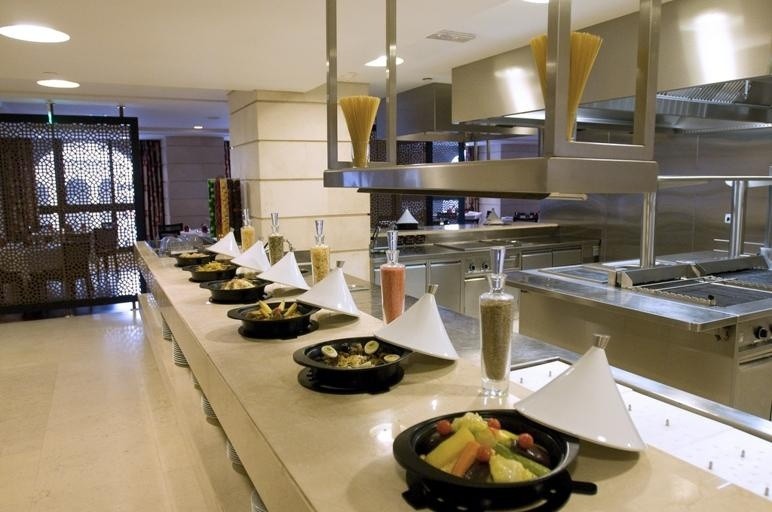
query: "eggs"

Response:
[365,340,380,354]
[321,345,338,357]
[383,355,400,362]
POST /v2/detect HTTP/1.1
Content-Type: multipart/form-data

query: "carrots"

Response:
[451,440,480,477]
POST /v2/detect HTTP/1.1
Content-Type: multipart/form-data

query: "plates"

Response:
[160,319,171,339]
[226,439,241,464]
[201,393,216,418]
[192,372,199,386]
[172,338,189,365]
[250,489,268,512]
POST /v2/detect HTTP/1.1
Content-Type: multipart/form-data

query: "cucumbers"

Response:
[496,440,551,477]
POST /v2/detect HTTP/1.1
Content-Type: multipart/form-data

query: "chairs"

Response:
[158,221,184,240]
[0,211,119,314]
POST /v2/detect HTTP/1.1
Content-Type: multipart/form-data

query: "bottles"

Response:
[380,228,406,324]
[205,176,242,245]
[479,247,513,398]
[514,211,534,220]
[240,203,330,287]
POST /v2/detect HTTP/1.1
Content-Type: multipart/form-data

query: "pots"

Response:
[172,250,322,339]
[292,335,418,385]
[394,409,580,499]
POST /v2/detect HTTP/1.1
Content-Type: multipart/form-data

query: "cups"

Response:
[566,112,576,142]
[439,216,444,227]
[397,234,426,246]
[350,141,371,168]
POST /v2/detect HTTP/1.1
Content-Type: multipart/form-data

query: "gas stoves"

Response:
[297,366,405,394]
[402,468,597,512]
